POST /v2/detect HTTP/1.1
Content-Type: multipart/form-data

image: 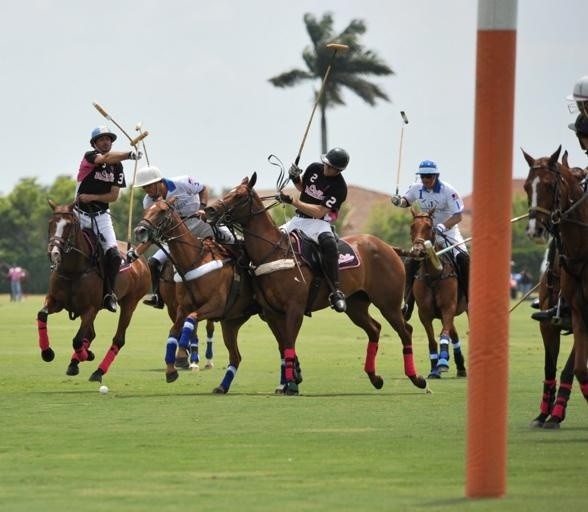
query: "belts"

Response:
[74,206,106,216]
[297,213,324,220]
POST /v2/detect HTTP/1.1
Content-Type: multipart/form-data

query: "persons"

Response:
[49,124,144,311]
[511,267,535,301]
[275,147,352,313]
[7,262,30,304]
[122,165,244,308]
[392,159,470,321]
[531,76,588,327]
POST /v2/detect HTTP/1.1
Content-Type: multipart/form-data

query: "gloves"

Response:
[127,249,140,263]
[288,162,302,184]
[129,151,142,160]
[274,192,293,204]
[432,223,447,233]
[391,194,401,206]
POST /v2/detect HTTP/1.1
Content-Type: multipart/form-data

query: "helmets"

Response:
[90,126,116,148]
[133,166,162,188]
[320,146,349,170]
[565,76,588,136]
[415,160,440,174]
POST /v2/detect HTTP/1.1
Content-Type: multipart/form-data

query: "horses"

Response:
[38,199,152,382]
[160,260,214,370]
[532,150,588,429]
[134,194,259,394]
[520,144,588,430]
[204,172,426,395]
[410,205,469,379]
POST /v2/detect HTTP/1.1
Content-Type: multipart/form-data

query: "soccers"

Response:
[100,386,109,394]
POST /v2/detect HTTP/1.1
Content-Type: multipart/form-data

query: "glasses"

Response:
[420,175,432,178]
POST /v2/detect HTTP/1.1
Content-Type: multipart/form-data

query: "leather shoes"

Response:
[532,303,571,321]
[531,298,539,308]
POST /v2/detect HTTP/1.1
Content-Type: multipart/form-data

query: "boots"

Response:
[457,252,469,304]
[144,258,164,309]
[103,247,121,312]
[318,232,347,312]
[402,260,420,321]
